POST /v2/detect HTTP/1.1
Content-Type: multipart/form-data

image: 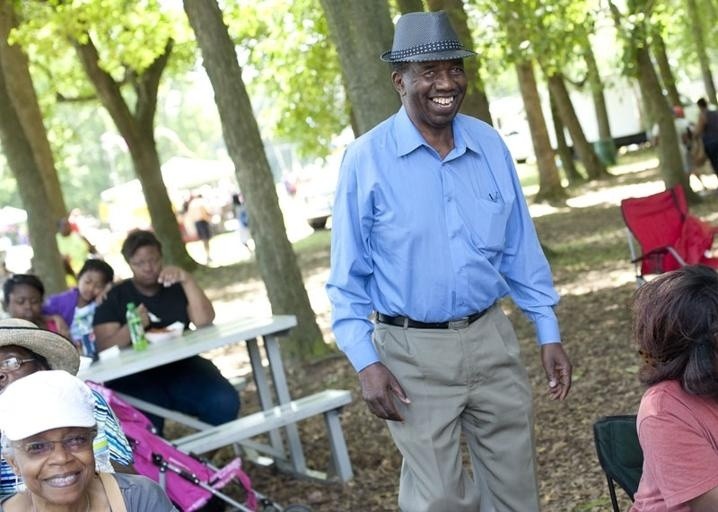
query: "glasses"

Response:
[0,354,41,370]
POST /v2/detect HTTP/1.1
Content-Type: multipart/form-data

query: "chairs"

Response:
[593,414,643,512]
[620,185,718,290]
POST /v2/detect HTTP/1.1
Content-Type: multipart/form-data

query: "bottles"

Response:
[77,313,100,360]
[124,302,151,351]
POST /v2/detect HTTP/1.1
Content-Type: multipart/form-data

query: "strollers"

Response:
[84,379,312,512]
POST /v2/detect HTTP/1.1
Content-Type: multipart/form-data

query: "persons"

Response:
[623,263,717,512]
[1,318,139,505]
[1,190,249,462]
[1,369,179,511]
[326,9,573,511]
[649,96,718,198]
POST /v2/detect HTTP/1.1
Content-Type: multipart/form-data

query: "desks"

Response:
[75,312,307,476]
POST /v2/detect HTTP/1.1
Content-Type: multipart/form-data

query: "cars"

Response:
[296,160,346,229]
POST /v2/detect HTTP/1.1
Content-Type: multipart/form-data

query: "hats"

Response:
[0,367,100,442]
[377,8,480,65]
[0,316,84,379]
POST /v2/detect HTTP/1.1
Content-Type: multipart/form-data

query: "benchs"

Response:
[166,388,355,486]
[225,377,274,468]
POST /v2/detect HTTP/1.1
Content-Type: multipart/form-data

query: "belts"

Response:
[372,300,501,331]
